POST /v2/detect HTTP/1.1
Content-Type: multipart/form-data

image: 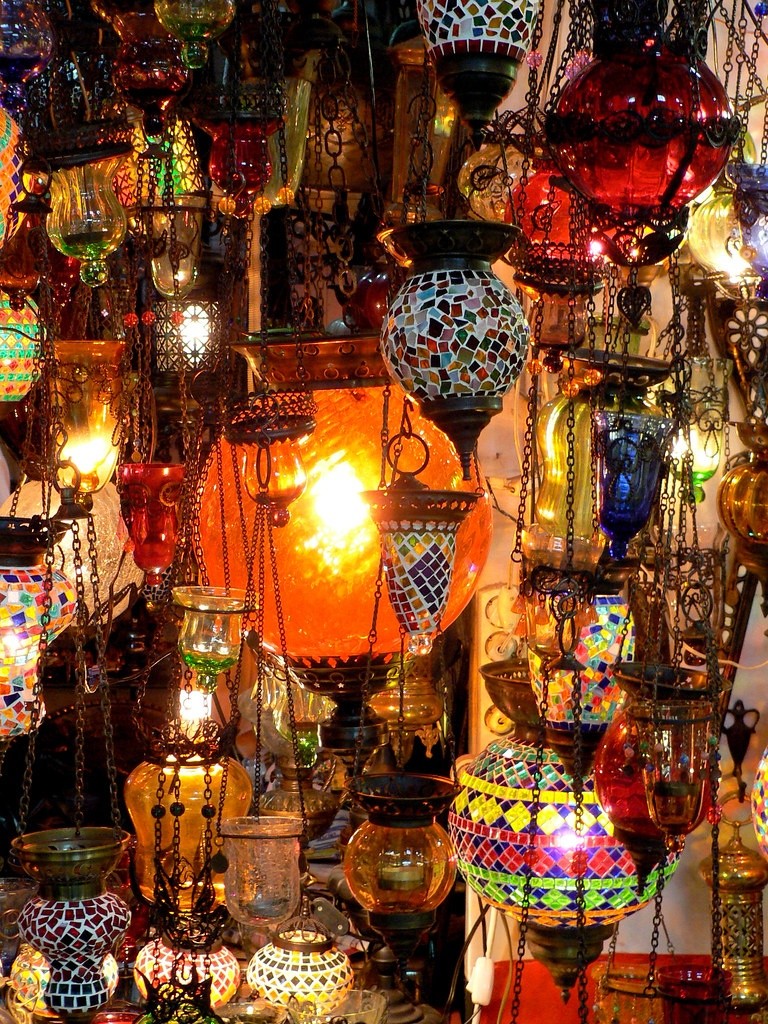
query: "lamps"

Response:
[1,0,768,1024]
